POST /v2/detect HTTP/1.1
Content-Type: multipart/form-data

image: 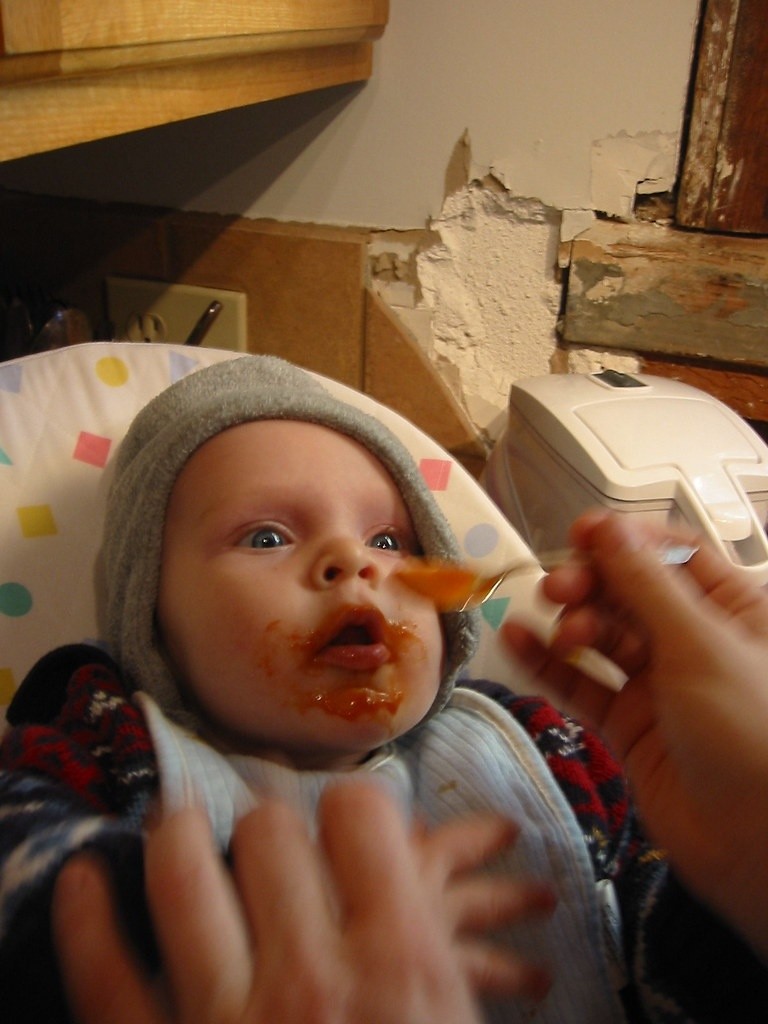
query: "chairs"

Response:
[1,342,597,748]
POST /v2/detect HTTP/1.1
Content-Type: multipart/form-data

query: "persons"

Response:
[0,352,767,1024]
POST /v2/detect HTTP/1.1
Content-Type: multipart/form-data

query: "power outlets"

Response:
[100,273,249,354]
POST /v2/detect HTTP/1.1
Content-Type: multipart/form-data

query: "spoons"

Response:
[388,542,699,612]
